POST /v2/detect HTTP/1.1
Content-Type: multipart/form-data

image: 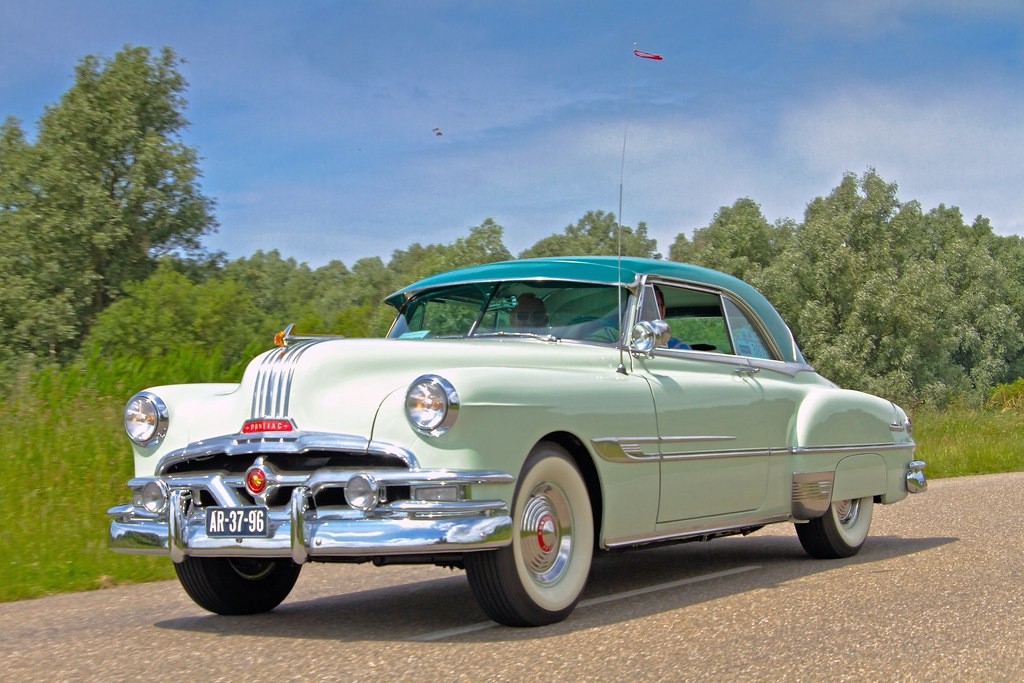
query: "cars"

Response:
[105,255,929,630]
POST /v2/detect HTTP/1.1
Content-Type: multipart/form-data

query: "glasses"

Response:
[516,310,546,321]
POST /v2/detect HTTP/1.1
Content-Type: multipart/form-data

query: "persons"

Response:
[509,290,549,328]
[615,282,691,352]
